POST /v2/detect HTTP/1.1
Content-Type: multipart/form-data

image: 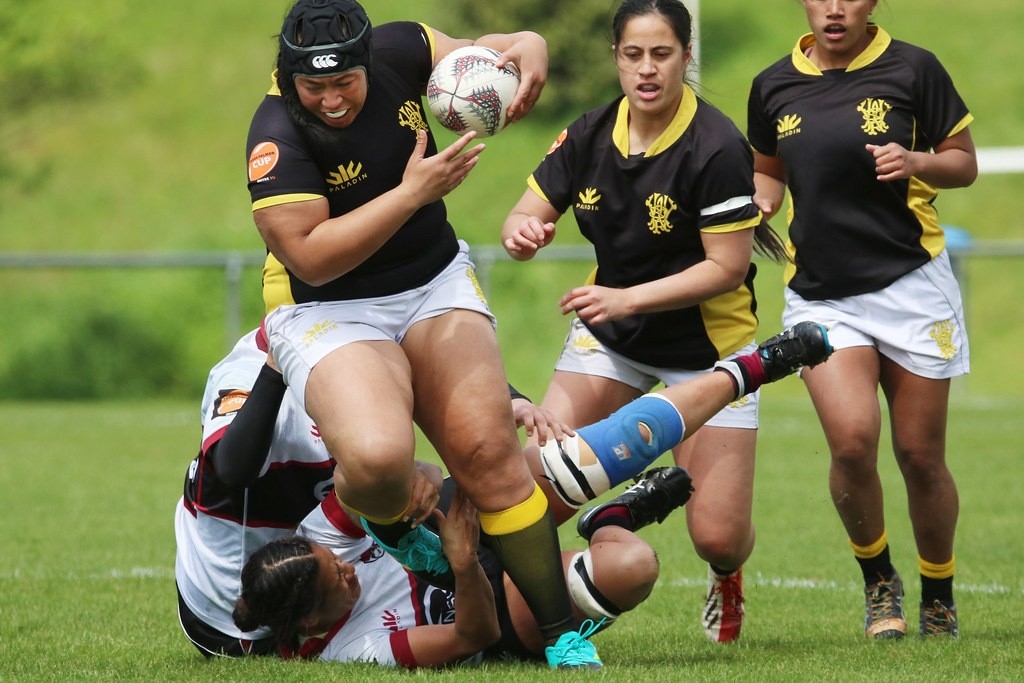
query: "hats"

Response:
[277,0,374,81]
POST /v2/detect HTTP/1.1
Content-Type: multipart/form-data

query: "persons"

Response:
[501,0,792,640]
[172,0,835,669]
[744,0,978,633]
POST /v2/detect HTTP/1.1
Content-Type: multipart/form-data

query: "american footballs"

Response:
[426,45,521,138]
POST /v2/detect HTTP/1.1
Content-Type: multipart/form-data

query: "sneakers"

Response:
[755,320,835,383]
[358,516,460,592]
[702,563,744,647]
[919,597,959,640]
[863,564,906,641]
[575,466,695,540]
[543,617,604,674]
